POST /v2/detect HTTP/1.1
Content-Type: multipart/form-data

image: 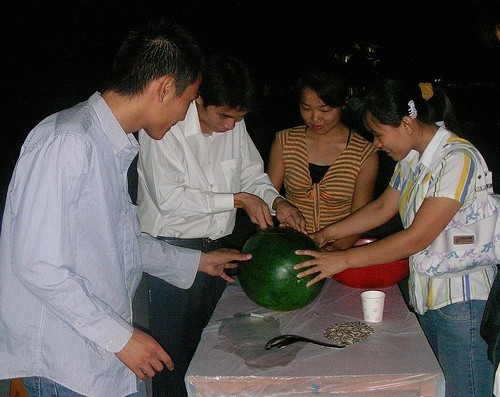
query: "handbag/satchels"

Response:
[407,144,500,279]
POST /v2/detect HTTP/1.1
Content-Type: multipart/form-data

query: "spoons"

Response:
[264,334,347,351]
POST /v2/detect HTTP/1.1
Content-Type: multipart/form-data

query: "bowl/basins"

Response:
[320,238,409,289]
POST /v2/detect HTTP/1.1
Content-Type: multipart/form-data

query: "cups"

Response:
[361,291,386,323]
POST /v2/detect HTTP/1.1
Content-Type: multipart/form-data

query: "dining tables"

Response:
[185,273,446,397]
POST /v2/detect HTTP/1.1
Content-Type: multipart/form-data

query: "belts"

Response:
[158,237,229,248]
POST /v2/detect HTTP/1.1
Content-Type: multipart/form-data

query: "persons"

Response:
[266,67,379,252]
[294,78,498,397]
[0,20,253,397]
[136,56,308,397]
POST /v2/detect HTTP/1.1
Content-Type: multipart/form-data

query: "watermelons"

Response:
[237,227,326,311]
